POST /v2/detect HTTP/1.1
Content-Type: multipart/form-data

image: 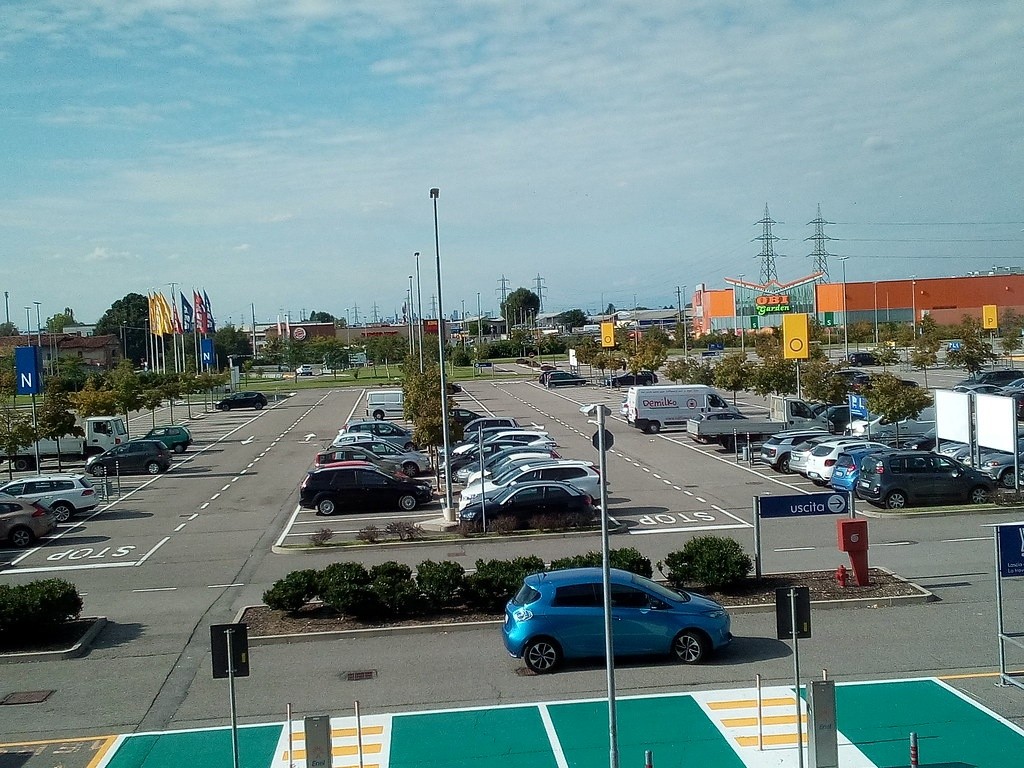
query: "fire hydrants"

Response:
[834,565,851,586]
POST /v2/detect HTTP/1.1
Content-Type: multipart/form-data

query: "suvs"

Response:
[0,472,101,547]
[215,391,269,410]
[296,364,313,376]
[298,408,600,532]
[607,369,658,387]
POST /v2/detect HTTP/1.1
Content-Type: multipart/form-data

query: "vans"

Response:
[366,391,407,419]
[627,384,740,434]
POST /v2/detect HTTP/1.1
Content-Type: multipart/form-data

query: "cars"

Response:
[500,567,731,673]
[760,352,1024,510]
[539,371,587,389]
[84,426,193,477]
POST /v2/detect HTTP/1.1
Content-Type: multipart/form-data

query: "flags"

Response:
[193,287,216,333]
[181,291,193,333]
[148,289,183,338]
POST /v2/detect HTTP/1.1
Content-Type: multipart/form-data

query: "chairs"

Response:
[374,426,380,435]
[908,458,917,469]
[56,481,65,490]
[24,483,37,494]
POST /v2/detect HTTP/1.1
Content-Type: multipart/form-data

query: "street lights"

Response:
[737,274,744,353]
[909,274,916,341]
[838,255,848,361]
[414,252,423,373]
[431,188,455,524]
[406,275,416,357]
[682,285,688,358]
[24,306,42,474]
[581,403,618,767]
[33,301,45,401]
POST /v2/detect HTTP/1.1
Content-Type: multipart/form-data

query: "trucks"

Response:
[0,415,129,470]
[683,394,835,451]
